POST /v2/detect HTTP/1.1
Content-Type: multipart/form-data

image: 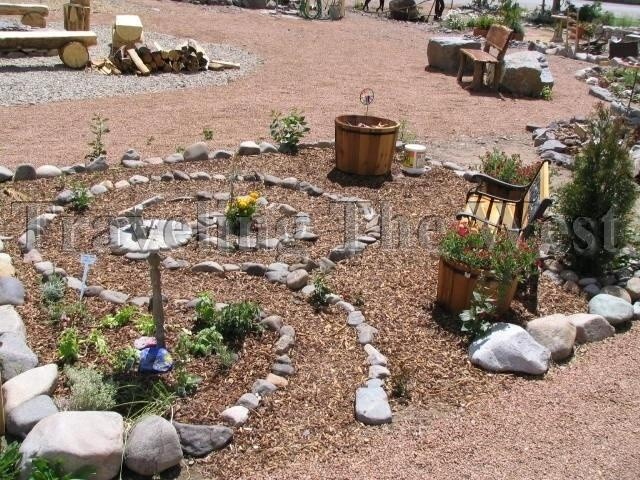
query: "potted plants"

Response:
[334,87,399,180]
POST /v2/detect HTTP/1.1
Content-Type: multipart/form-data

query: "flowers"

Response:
[473,148,543,192]
[436,217,545,289]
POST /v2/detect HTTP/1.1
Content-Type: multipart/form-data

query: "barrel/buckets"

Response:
[402,144,431,174]
[334,115,399,179]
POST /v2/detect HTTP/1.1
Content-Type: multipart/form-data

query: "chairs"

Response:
[565,4,589,57]
[456,23,514,95]
[460,158,552,242]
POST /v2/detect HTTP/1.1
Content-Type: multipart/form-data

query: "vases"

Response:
[483,186,527,201]
